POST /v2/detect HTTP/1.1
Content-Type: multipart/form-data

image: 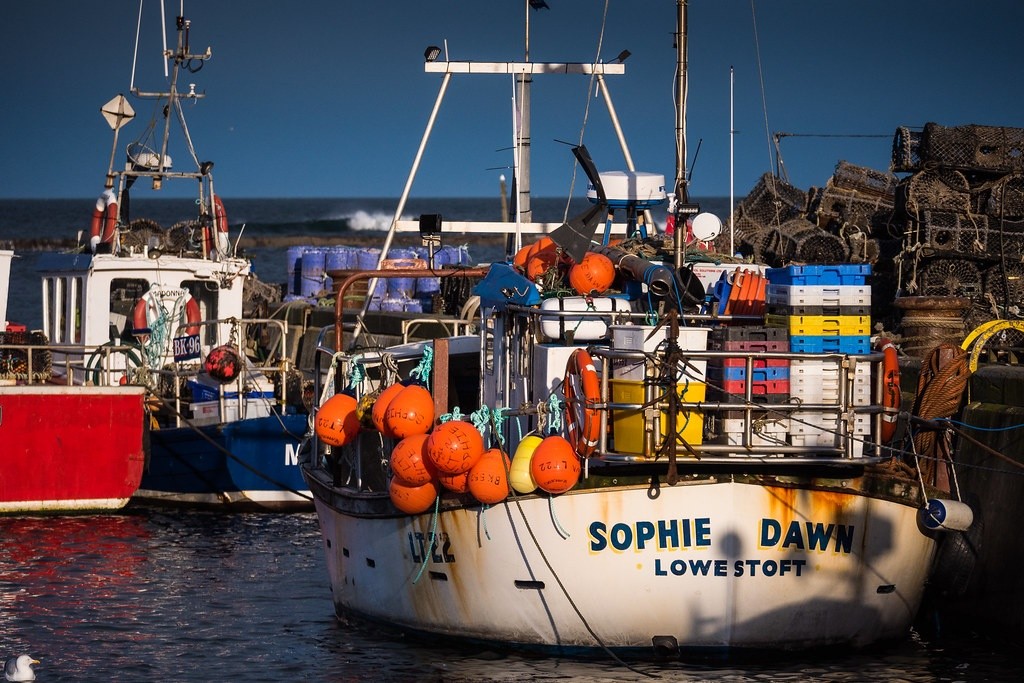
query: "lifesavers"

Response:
[871,336,901,443]
[562,348,601,460]
[133,284,202,358]
[204,195,229,260]
[89,190,118,254]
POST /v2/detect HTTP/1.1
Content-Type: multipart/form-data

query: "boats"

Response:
[298,0,976,663]
[34,1,314,513]
[1,249,146,513]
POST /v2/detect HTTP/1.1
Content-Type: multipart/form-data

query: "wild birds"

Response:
[4,654,40,683]
